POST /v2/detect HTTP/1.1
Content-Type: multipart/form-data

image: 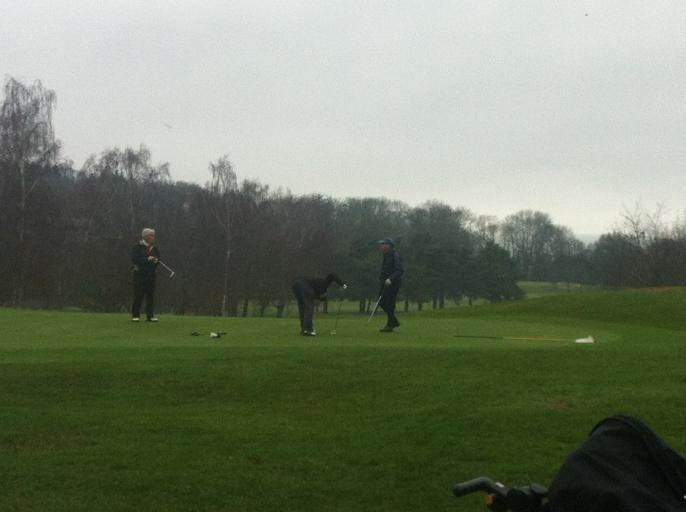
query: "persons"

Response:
[375,237,405,333]
[127,227,164,323]
[290,270,348,337]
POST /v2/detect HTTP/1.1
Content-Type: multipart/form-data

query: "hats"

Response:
[143,228,154,237]
[379,237,395,245]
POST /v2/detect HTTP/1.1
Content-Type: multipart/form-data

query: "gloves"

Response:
[385,278,392,287]
[343,284,347,290]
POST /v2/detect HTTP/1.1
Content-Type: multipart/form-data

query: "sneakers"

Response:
[146,318,158,322]
[300,328,317,336]
[379,320,400,332]
[132,317,139,321]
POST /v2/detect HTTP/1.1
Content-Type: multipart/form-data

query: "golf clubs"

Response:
[367,284,387,323]
[159,260,174,278]
[330,288,346,335]
